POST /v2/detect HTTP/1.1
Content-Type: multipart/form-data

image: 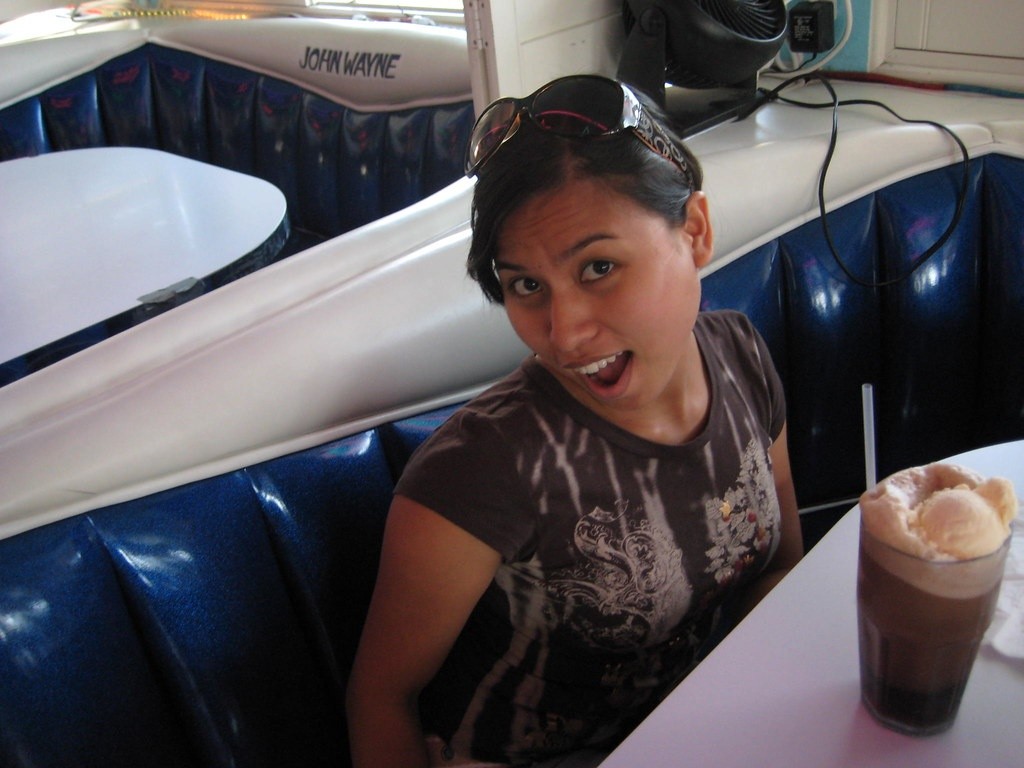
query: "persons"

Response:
[345,73,805,768]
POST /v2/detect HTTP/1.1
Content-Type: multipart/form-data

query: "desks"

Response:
[0,145,292,388]
[597,438,1024,768]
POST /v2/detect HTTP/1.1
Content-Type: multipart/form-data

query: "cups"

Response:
[856,512,1013,737]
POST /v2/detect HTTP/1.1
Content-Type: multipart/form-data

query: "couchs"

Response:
[0,8,1024,768]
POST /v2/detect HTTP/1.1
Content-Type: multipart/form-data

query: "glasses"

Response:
[464,75,694,192]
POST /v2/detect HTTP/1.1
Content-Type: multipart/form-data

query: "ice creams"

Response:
[859,463,1017,600]
